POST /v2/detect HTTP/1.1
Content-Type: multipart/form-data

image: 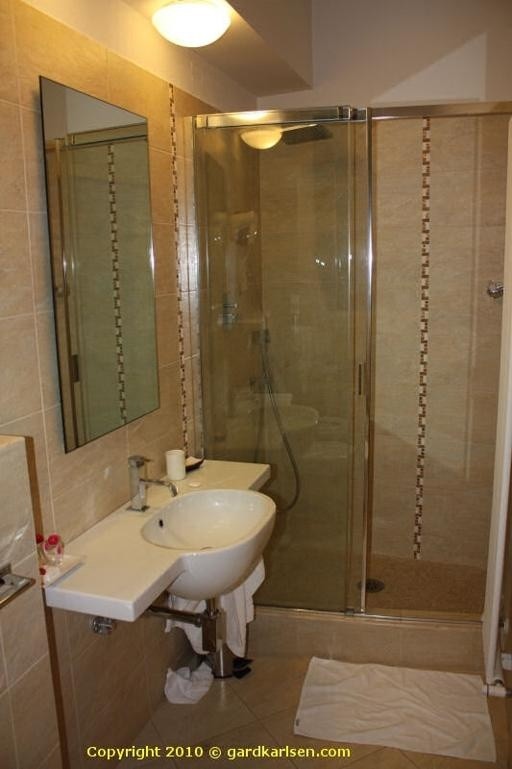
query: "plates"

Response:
[185,455,206,472]
[42,552,86,589]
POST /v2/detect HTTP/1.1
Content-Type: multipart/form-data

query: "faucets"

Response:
[128,453,178,512]
[226,389,253,415]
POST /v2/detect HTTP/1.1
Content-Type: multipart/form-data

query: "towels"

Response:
[163,554,270,661]
[293,656,501,761]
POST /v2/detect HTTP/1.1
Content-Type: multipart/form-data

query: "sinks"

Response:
[140,489,277,601]
[249,403,321,435]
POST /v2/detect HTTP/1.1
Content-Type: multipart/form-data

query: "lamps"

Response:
[148,3,236,50]
[240,124,283,152]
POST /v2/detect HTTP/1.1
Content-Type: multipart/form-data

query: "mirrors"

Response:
[37,72,161,454]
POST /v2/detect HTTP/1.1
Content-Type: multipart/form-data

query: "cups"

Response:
[165,448,186,483]
